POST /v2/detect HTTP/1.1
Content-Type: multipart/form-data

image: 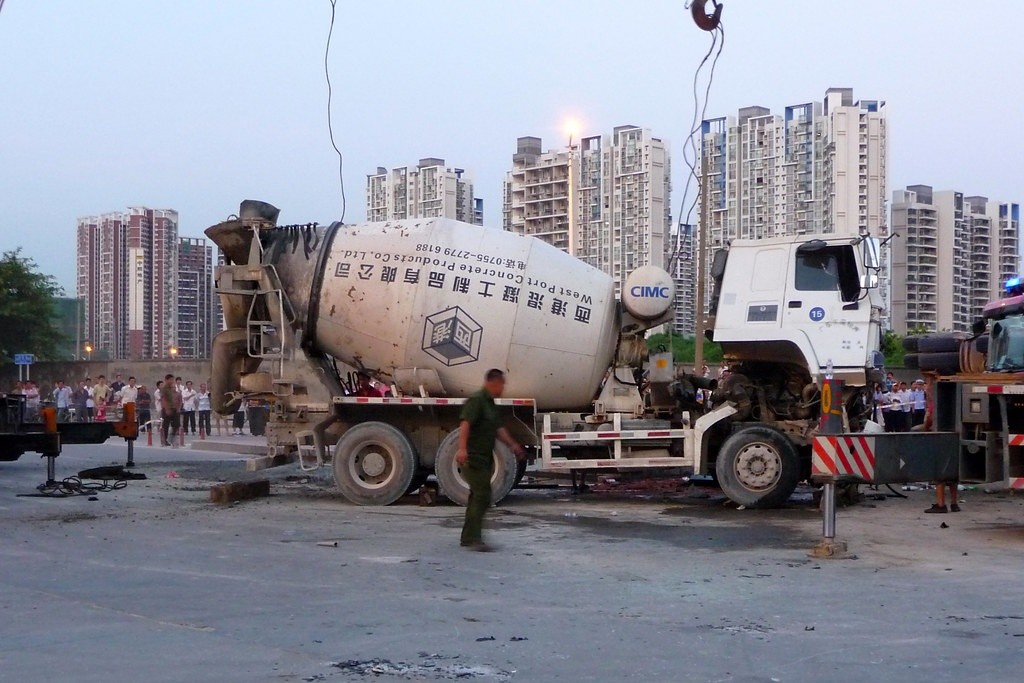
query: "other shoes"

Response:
[239,432,244,435]
[471,543,491,551]
[461,542,483,546]
[232,432,238,435]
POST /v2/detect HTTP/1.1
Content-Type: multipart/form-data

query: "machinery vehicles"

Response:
[971,278,1024,478]
[205,200,885,512]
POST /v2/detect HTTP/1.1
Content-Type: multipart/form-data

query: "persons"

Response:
[454,368,524,551]
[691,356,733,406]
[72,381,89,422]
[10,381,40,423]
[136,385,151,434]
[863,371,928,432]
[182,381,198,436]
[113,376,138,412]
[153,374,185,448]
[83,378,94,421]
[51,378,73,423]
[110,375,126,391]
[232,399,246,435]
[918,371,961,513]
[92,374,112,422]
[194,383,212,436]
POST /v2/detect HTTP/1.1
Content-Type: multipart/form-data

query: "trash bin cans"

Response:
[247,405,271,436]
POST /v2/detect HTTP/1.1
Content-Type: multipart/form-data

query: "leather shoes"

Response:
[951,504,960,512]
[924,503,947,513]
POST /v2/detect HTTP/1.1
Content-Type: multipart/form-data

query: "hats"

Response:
[916,380,925,384]
